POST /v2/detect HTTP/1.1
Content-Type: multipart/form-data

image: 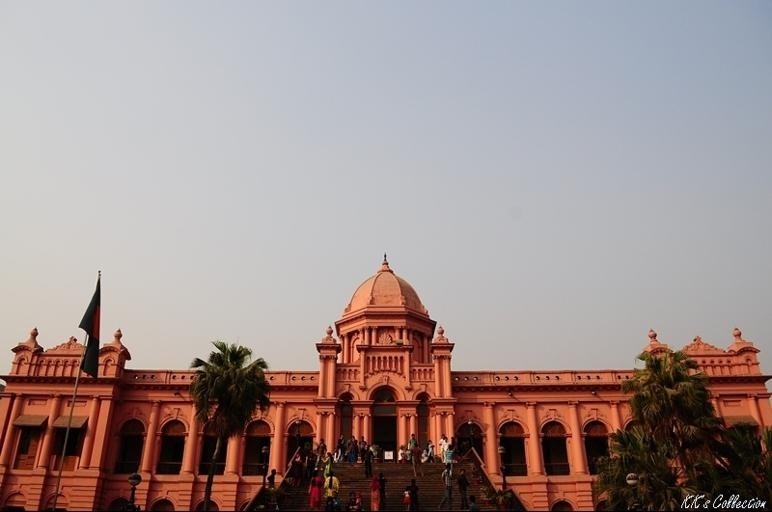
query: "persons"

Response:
[267,432,478,512]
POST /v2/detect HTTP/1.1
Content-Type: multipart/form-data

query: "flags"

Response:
[78,278,100,380]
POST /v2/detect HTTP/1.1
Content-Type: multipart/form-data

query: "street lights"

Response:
[261,446,269,488]
[126,473,141,512]
[498,447,506,490]
[295,420,301,446]
[626,473,642,512]
[468,419,474,447]
